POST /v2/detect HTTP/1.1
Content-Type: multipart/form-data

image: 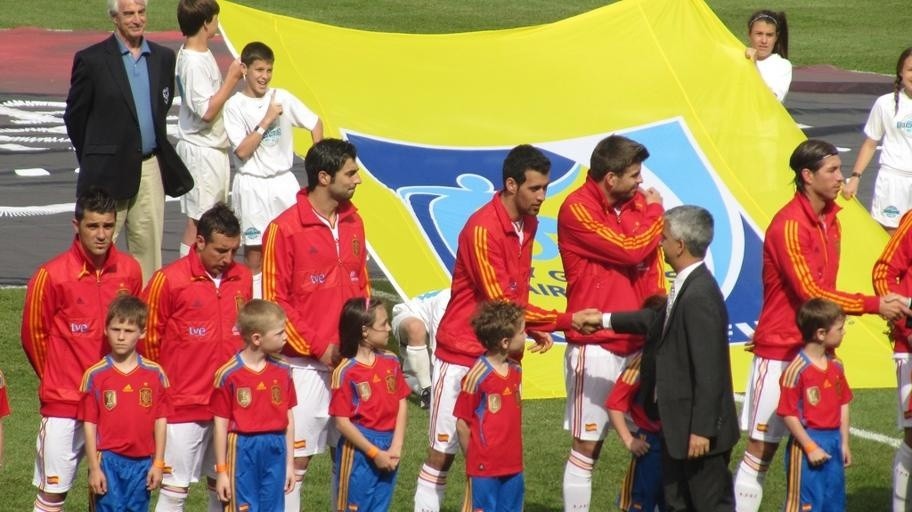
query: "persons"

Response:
[262,138,411,512]
[839,46,912,240]
[734,140,911,512]
[744,10,793,104]
[22,187,298,512]
[389,135,741,511]
[62,0,323,297]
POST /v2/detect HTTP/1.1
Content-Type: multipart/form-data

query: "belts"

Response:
[142,148,156,162]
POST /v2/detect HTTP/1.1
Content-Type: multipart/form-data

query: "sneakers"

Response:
[420,386,432,409]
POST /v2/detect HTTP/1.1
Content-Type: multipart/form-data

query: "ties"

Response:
[664,284,675,326]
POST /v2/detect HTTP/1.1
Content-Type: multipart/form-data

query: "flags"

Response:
[212,0,896,399]
